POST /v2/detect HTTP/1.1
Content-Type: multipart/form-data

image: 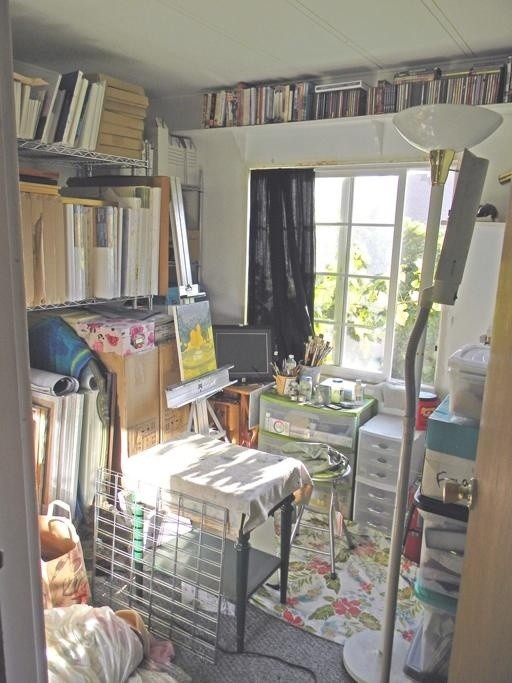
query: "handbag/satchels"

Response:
[39,514,92,606]
[280,442,350,475]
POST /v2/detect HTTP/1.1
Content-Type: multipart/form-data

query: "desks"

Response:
[226,384,268,451]
[121,431,302,654]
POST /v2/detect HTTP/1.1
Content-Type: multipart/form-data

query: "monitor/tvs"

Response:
[211,324,276,385]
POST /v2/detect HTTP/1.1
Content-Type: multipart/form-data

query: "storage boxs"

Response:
[420,394,480,508]
[353,412,429,534]
[414,485,469,599]
[448,343,490,420]
[93,348,158,456]
[157,339,189,443]
[259,390,379,522]
[413,576,457,675]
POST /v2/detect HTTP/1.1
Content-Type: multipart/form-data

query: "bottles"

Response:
[284,353,296,372]
[300,376,363,405]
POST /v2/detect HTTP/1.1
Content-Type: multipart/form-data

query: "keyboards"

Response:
[208,392,242,405]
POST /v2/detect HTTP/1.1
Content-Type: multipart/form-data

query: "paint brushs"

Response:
[304,334,333,367]
[269,358,302,377]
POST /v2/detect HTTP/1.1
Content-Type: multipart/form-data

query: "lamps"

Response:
[341,101,504,682]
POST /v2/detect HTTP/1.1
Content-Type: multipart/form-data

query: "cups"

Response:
[299,360,320,384]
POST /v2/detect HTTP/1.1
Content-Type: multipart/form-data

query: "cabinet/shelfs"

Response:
[15,136,155,314]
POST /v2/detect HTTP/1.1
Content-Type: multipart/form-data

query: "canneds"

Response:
[299,375,312,401]
[331,380,344,403]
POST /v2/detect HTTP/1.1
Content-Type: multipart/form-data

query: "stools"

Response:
[289,462,356,581]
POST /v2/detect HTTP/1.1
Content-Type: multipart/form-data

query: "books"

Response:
[18,165,170,308]
[200,56,511,129]
[419,520,467,600]
[12,60,149,161]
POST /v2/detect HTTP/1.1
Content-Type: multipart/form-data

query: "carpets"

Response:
[249,507,432,645]
